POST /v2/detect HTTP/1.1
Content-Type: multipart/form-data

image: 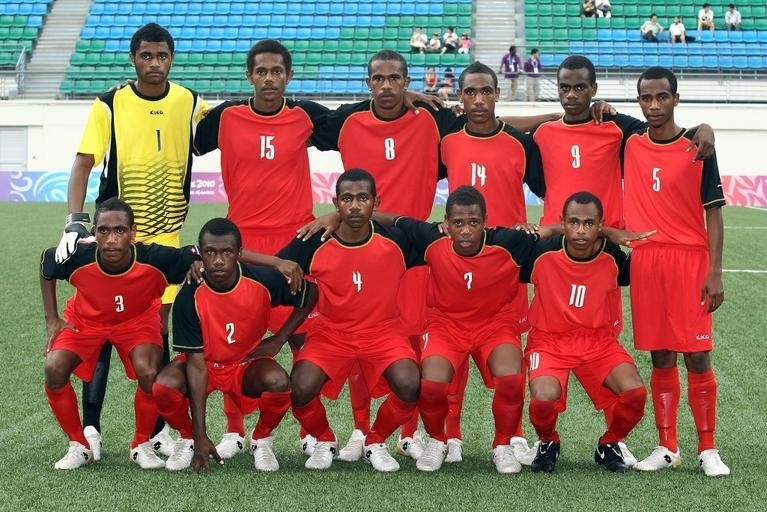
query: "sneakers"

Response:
[248,428,280,471]
[82,426,102,461]
[150,430,176,456]
[510,436,530,462]
[519,441,543,466]
[618,442,637,467]
[300,434,317,456]
[632,445,680,470]
[305,431,338,469]
[165,438,194,471]
[531,441,560,473]
[698,448,730,478]
[416,437,447,471]
[491,445,522,474]
[595,436,629,474]
[337,429,367,462]
[129,441,165,470]
[52,442,94,470]
[396,430,425,460]
[445,438,464,463]
[362,435,400,472]
[209,433,248,459]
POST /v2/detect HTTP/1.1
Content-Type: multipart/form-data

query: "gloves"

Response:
[54,212,97,266]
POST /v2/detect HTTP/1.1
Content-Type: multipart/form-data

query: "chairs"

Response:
[523,1,766,76]
[0,0,49,69]
[59,1,474,100]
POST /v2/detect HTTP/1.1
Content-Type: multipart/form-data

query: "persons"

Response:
[434,191,649,474]
[581,1,612,18]
[40,196,307,470]
[296,179,661,475]
[432,57,547,463]
[449,56,716,466]
[499,45,522,100]
[178,167,539,473]
[410,27,471,55]
[669,16,686,43]
[696,3,716,40]
[106,40,446,459]
[524,49,541,100]
[46,24,219,461]
[151,214,319,473]
[724,3,742,31]
[303,48,562,464]
[425,65,455,100]
[640,14,664,43]
[620,67,731,478]
